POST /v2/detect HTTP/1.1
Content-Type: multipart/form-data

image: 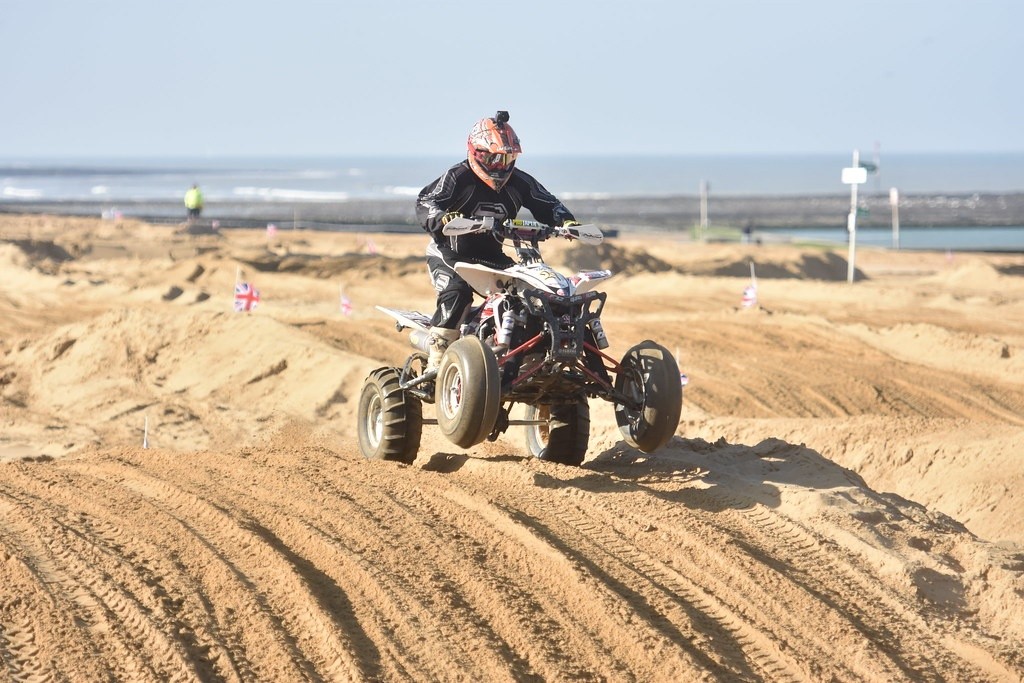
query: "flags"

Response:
[341,299,354,316]
[268,224,277,236]
[234,284,260,313]
[739,286,756,306]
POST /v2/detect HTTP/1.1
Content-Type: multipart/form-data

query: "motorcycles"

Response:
[357,209,684,467]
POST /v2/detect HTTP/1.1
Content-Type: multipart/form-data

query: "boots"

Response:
[417,326,460,394]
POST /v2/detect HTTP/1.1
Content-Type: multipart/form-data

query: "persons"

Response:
[415,111,583,374]
[184,184,203,220]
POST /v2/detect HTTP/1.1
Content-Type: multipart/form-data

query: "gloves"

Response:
[562,220,583,241]
[442,211,466,225]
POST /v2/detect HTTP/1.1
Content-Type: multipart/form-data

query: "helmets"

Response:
[467,110,523,194]
